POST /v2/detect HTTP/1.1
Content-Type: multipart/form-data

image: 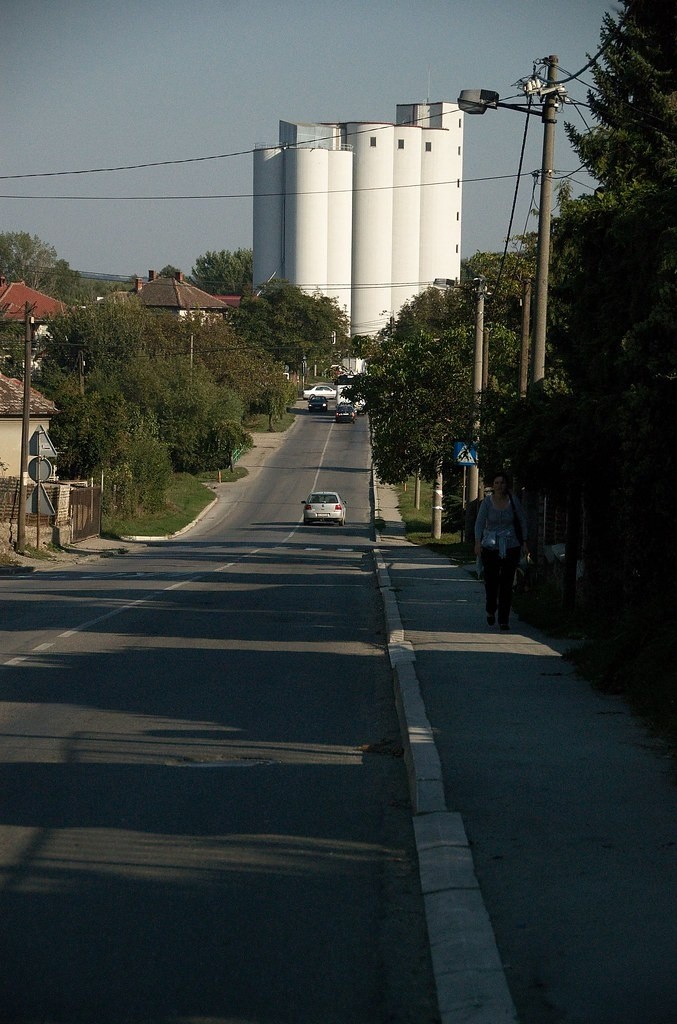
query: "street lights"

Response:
[433,277,484,557]
[459,89,559,561]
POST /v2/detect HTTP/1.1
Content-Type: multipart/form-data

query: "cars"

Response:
[303,386,336,399]
[301,491,347,526]
[336,405,354,423]
[309,396,328,414]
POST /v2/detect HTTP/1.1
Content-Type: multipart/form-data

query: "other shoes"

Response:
[487,615,495,625]
[500,623,510,631]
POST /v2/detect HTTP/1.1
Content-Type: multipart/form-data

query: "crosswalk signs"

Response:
[455,441,476,465]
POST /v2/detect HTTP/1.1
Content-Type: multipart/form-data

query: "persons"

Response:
[474,470,529,632]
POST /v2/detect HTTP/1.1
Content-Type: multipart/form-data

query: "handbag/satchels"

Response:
[509,494,524,547]
